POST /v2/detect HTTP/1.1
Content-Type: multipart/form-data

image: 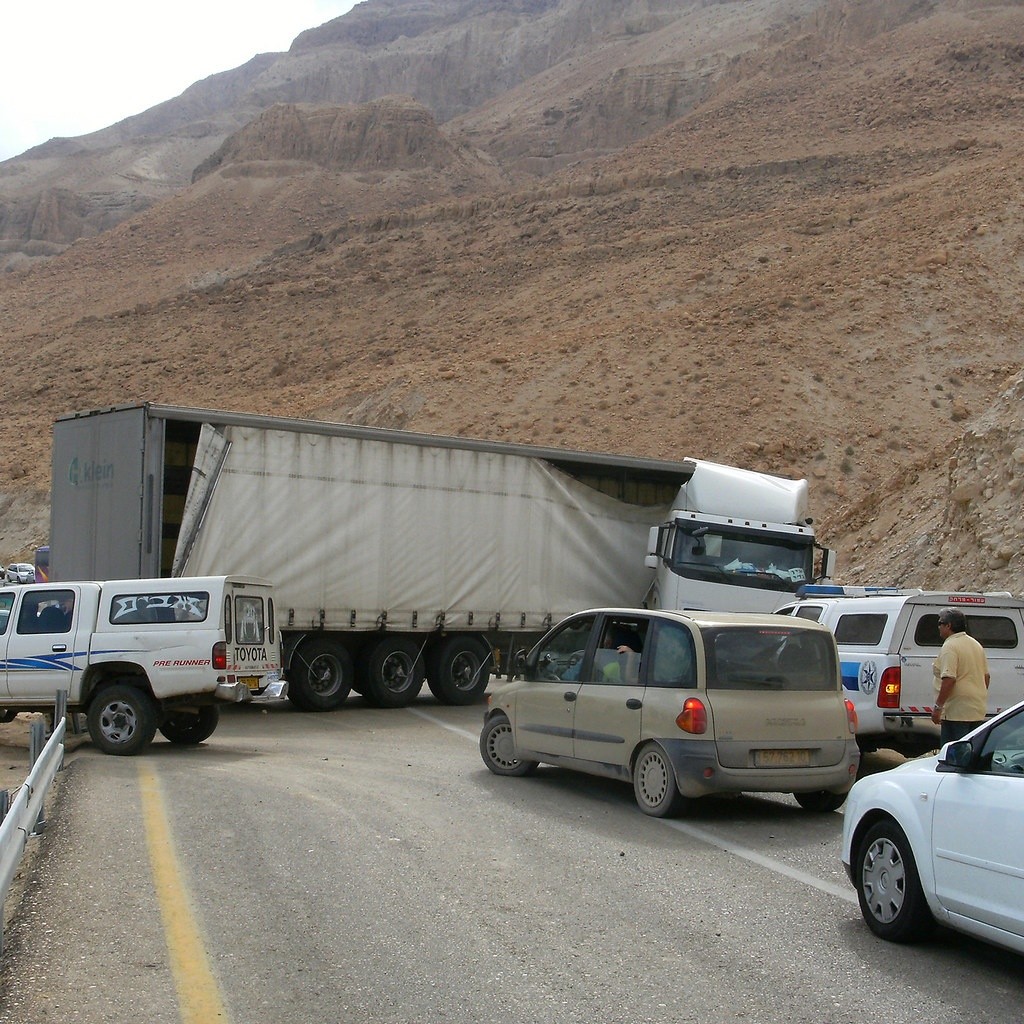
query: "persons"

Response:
[559,626,644,685]
[931,607,990,748]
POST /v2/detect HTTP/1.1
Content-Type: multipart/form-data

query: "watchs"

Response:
[934,704,943,711]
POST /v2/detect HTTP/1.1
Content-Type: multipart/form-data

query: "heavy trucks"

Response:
[42,399,835,714]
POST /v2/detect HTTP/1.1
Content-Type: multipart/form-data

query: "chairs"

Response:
[612,624,640,653]
[38,605,64,632]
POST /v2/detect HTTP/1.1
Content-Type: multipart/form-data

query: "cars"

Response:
[477,605,861,824]
[0,562,38,586]
[841,698,1024,971]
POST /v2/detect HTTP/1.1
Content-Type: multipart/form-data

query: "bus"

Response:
[34,544,50,585]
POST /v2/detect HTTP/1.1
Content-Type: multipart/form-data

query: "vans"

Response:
[765,583,1024,757]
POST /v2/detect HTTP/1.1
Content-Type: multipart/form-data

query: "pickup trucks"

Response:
[0,574,292,756]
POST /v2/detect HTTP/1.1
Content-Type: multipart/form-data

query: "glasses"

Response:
[937,620,947,626]
